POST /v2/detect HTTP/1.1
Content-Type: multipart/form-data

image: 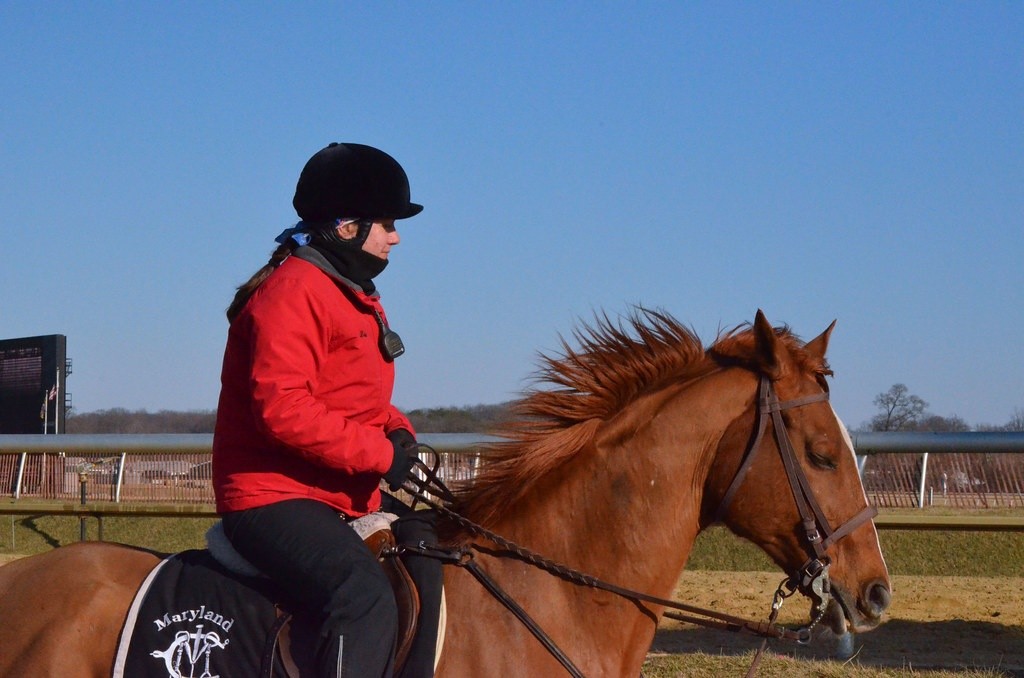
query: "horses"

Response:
[0,308,892,678]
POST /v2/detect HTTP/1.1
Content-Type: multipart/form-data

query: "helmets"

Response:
[293,142,424,228]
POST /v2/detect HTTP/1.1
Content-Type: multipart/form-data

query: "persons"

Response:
[211,141,424,678]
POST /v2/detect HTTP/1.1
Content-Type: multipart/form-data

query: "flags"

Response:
[40,397,46,418]
[49,374,56,400]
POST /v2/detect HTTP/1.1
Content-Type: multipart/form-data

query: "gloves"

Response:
[382,440,412,492]
[386,427,419,462]
